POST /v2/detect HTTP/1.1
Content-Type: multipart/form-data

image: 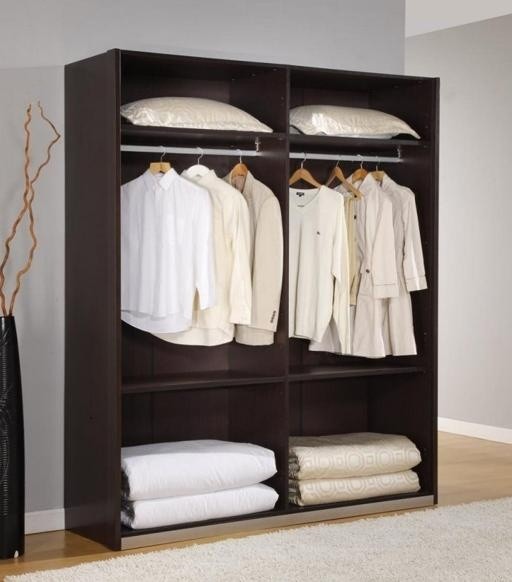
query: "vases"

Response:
[0,312,28,560]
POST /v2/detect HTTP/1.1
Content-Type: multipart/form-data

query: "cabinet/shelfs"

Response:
[63,48,441,551]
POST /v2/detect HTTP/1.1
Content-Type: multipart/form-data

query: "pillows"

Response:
[291,104,420,139]
[120,97,274,134]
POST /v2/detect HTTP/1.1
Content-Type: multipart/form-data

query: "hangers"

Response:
[372,156,385,181]
[149,143,172,175]
[185,145,209,175]
[229,149,250,178]
[352,154,369,182]
[325,154,364,198]
[289,152,321,187]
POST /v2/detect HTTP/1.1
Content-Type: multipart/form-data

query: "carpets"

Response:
[2,498,510,581]
